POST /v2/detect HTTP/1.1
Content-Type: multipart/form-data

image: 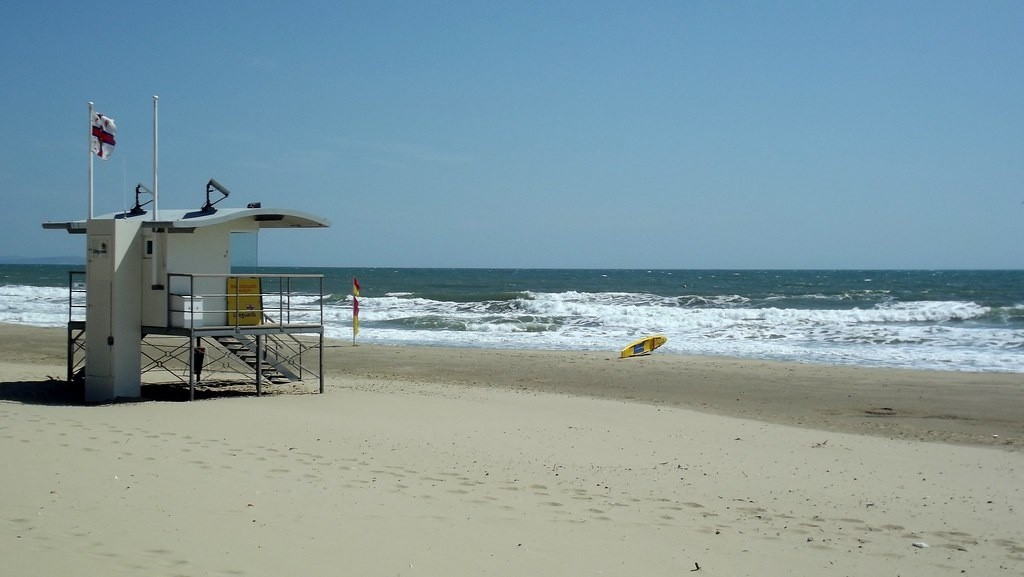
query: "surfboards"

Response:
[621,335,668,356]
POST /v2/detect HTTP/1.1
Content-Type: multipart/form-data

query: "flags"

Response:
[90,112,116,161]
